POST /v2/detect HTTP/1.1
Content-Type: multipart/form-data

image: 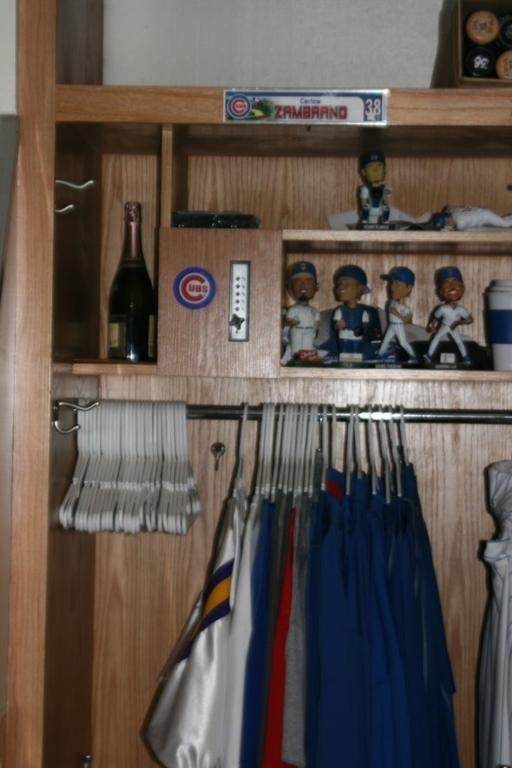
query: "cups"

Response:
[486,279,512,370]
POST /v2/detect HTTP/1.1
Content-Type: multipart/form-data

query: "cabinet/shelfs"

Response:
[1,1,512,768]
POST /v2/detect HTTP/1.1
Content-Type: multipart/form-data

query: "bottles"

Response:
[106,200,158,364]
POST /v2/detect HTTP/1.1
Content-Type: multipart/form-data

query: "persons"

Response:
[421,266,474,366]
[431,204,511,231]
[372,266,420,364]
[355,145,433,230]
[285,260,321,359]
[331,265,379,354]
